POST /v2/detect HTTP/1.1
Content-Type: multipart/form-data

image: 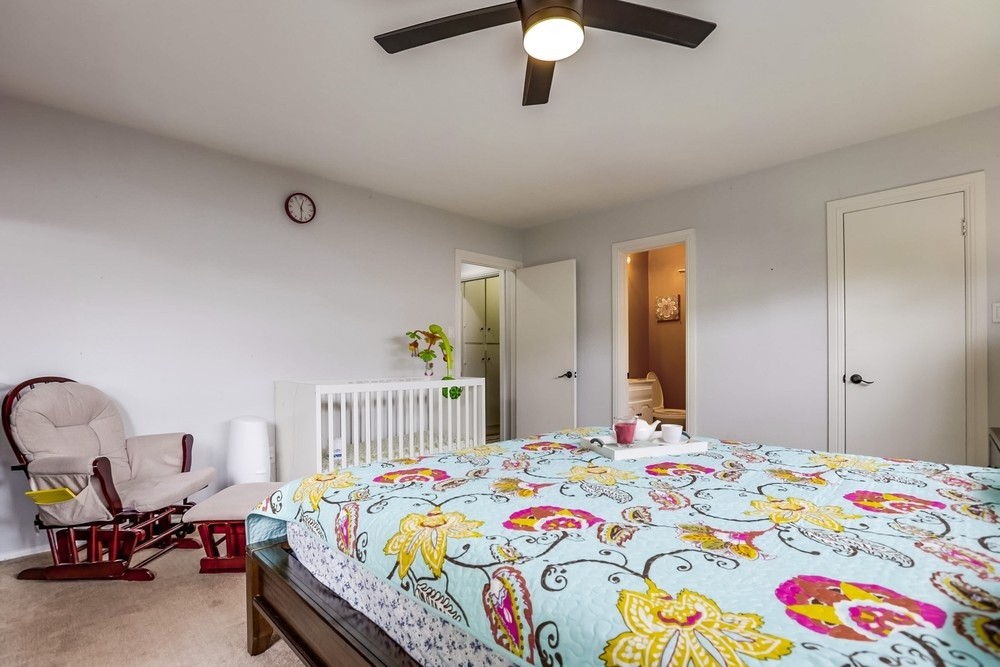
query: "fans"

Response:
[374,0,717,108]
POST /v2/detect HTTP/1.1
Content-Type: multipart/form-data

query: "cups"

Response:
[614,416,637,447]
[661,424,683,443]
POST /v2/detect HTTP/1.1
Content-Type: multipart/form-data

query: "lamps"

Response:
[522,8,584,61]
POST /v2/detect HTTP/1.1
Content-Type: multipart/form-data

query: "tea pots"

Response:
[612,416,662,441]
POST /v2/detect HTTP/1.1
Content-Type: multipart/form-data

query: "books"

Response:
[25,487,76,503]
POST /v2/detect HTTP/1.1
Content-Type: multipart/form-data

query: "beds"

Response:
[244,425,1000,667]
[275,377,486,485]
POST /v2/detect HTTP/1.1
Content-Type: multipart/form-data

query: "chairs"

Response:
[2,377,203,582]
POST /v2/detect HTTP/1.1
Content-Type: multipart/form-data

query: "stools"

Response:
[182,481,284,574]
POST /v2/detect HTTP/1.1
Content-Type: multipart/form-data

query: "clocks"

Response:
[285,192,317,226]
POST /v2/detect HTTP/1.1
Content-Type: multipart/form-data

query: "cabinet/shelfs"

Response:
[630,386,653,425]
[464,343,500,427]
[462,275,500,344]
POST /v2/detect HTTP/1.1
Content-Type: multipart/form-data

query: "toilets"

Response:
[646,371,687,430]
